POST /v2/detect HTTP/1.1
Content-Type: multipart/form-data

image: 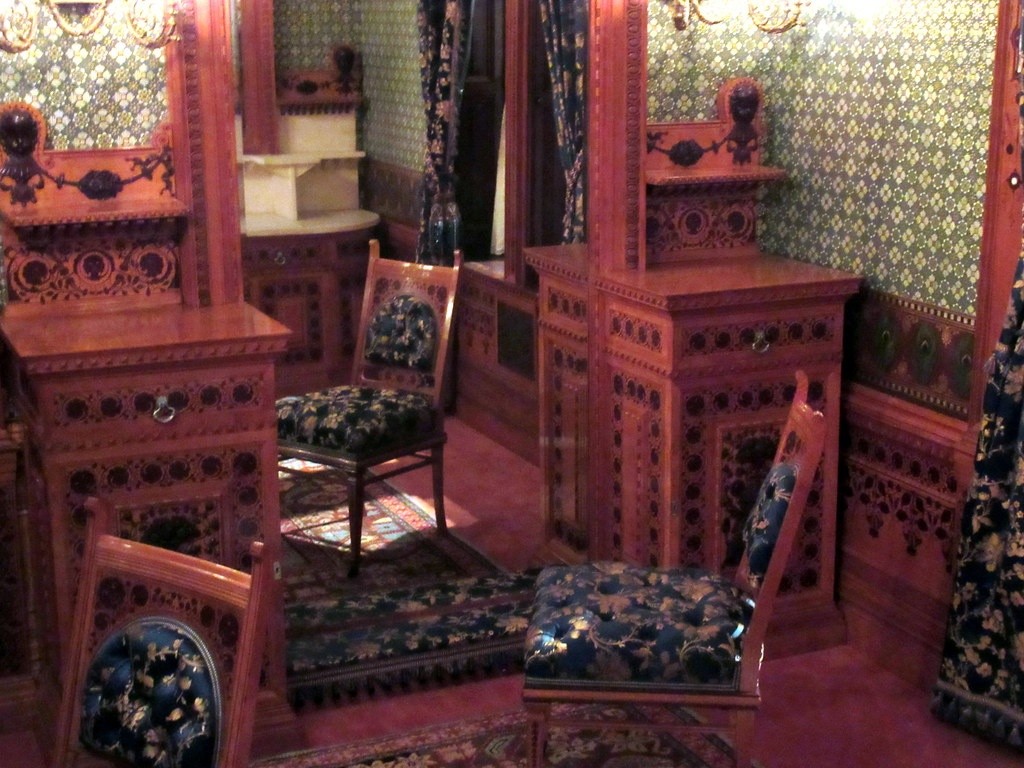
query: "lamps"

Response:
[0,0,182,55]
[666,0,811,34]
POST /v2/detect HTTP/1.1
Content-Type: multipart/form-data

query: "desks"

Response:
[236,208,381,389]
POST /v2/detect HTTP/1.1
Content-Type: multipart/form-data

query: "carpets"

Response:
[279,452,536,715]
[245,703,766,768]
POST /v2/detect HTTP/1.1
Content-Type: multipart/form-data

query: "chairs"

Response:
[49,496,275,768]
[519,371,830,768]
[275,240,466,581]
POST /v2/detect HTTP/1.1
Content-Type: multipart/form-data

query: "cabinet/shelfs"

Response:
[0,305,311,764]
[522,78,866,663]
